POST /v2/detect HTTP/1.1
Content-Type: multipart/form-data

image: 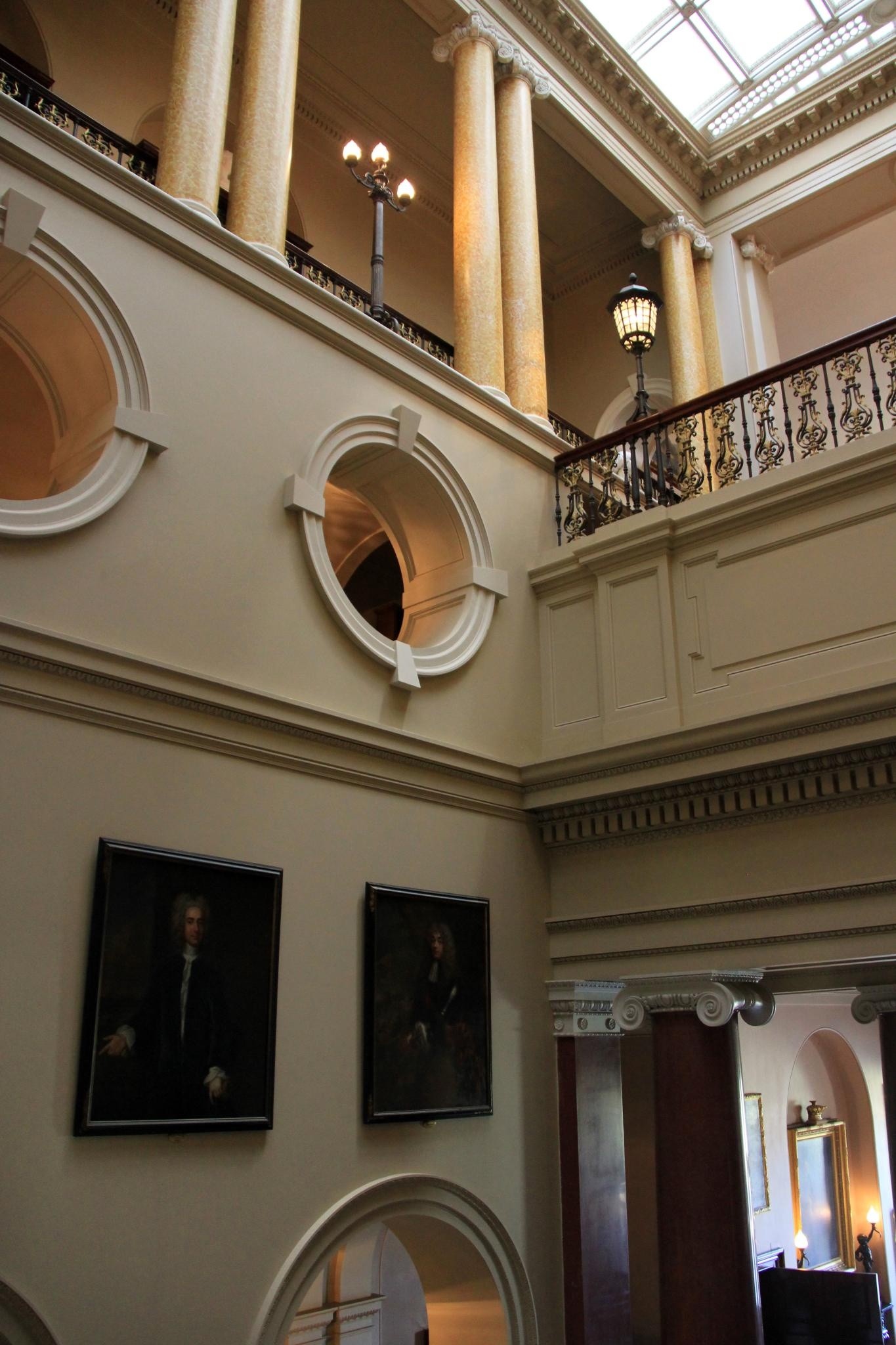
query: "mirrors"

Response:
[787,1101,856,1272]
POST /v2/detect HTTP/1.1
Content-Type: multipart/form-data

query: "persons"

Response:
[855,1227,877,1273]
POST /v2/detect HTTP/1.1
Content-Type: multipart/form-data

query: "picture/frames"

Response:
[72,837,284,1139]
[744,1093,771,1216]
[363,882,494,1130]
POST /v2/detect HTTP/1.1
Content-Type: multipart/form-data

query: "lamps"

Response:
[606,273,669,517]
[342,140,416,334]
[795,1229,810,1271]
[854,1205,881,1273]
